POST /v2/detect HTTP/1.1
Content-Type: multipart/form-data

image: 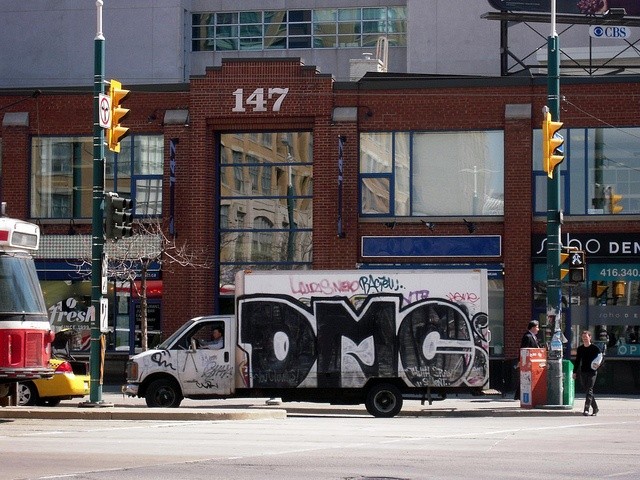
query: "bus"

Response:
[0,200,54,381]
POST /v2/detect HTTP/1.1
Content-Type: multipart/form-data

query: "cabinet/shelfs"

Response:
[589,167,640,213]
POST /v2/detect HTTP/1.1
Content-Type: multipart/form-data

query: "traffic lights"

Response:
[560,253,569,282]
[108,80,131,155]
[542,106,564,179]
[597,281,609,299]
[106,192,133,242]
[569,250,586,284]
[609,186,624,215]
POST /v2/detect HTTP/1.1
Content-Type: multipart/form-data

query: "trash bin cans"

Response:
[69,355,91,375]
[546,360,575,406]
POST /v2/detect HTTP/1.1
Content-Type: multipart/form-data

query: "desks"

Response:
[349,59,384,82]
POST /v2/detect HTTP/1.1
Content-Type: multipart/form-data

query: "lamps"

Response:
[386,221,399,228]
[421,220,435,231]
[3,110,31,127]
[462,219,474,233]
[148,108,190,128]
[330,107,372,127]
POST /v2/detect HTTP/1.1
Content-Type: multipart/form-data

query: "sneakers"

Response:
[590,409,599,416]
[583,410,588,415]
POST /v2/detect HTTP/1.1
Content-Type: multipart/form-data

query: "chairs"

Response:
[376,36,388,72]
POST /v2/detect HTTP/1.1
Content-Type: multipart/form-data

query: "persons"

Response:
[572,331,603,416]
[514,320,540,401]
[201,327,223,350]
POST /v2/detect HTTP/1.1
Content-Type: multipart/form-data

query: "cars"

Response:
[15,326,90,406]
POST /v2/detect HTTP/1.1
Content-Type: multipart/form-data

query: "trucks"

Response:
[120,268,493,417]
